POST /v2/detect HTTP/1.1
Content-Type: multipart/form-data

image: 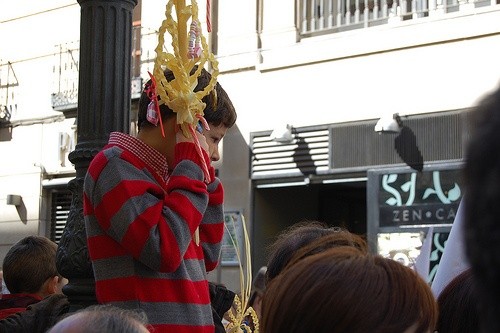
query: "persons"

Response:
[85,64,237,333]
[209,221,438,333]
[0,235,152,333]
[435,85,500,333]
